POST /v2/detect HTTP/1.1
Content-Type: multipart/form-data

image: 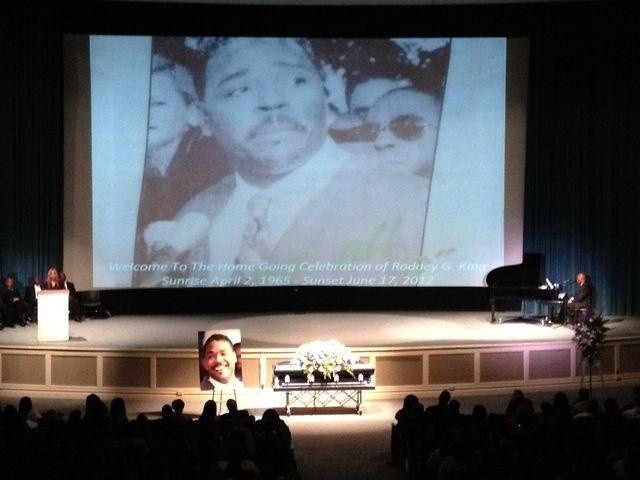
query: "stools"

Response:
[572,307,588,326]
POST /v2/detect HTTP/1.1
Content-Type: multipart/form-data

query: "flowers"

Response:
[572,310,611,368]
[288,338,356,383]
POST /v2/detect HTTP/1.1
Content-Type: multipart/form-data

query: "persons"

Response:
[201,334,243,389]
[1,267,86,329]
[389,388,640,480]
[135,36,455,286]
[556,272,595,325]
[1,394,301,479]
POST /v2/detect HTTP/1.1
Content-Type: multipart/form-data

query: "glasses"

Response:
[354,113,438,142]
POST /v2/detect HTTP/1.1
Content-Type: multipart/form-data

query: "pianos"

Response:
[486,252,568,327]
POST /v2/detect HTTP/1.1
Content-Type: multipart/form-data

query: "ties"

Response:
[228,202,274,285]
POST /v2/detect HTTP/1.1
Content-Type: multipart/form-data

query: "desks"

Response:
[272,377,376,414]
